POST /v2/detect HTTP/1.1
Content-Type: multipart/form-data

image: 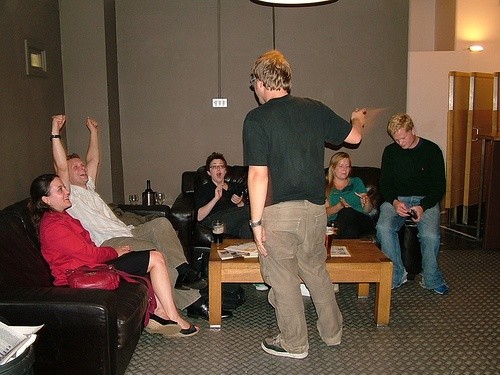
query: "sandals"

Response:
[144,313,181,334]
[163,325,200,337]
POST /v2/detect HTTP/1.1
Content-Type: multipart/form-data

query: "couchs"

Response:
[0,194,170,375]
[170,164,422,282]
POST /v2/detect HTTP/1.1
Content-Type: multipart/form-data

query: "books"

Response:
[217,241,259,260]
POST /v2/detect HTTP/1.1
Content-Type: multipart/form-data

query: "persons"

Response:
[192,151,252,247]
[27,174,200,338]
[52,115,207,311]
[375,113,449,295]
[325,151,374,241]
[242,51,367,359]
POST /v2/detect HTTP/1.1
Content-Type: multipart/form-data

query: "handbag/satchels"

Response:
[65,264,119,291]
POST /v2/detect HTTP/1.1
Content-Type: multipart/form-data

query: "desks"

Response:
[210,240,393,327]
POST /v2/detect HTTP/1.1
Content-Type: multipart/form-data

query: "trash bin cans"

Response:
[0,343,35,375]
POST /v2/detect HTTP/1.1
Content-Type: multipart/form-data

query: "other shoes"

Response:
[419,276,449,295]
[299,283,310,297]
[391,275,407,290]
[252,284,268,291]
[332,283,340,293]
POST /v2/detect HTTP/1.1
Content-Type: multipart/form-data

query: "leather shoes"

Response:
[187,298,233,320]
[174,268,209,290]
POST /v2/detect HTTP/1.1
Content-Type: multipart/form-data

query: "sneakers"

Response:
[261,334,308,359]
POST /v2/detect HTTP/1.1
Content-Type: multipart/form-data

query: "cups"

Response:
[129,194,139,205]
[324,220,335,262]
[154,192,165,206]
[212,220,224,245]
[142,192,155,207]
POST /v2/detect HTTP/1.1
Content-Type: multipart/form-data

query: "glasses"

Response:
[209,165,225,169]
[249,76,257,86]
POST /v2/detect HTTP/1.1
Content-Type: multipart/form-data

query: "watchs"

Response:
[249,220,261,227]
[49,134,61,141]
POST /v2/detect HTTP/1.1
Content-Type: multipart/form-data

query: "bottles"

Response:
[144,180,153,194]
